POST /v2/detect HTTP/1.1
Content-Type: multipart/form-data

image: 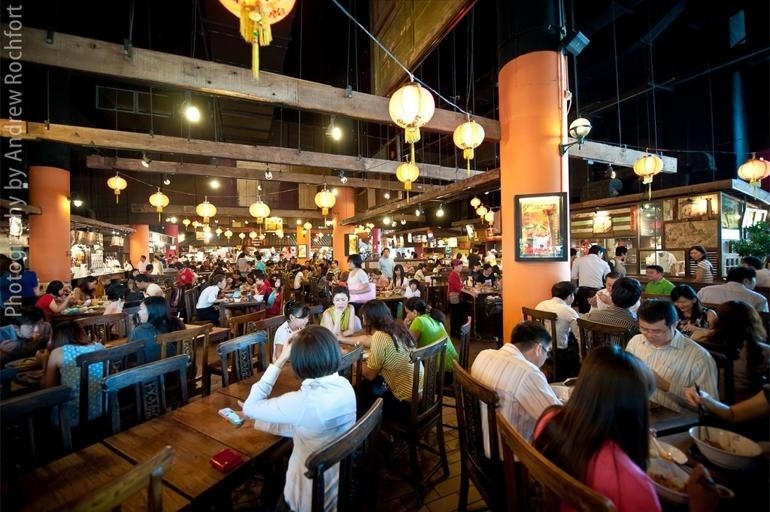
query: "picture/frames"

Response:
[513,193,567,262]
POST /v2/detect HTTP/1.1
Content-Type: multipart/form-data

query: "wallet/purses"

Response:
[209,449,243,474]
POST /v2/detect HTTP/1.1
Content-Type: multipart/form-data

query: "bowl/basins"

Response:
[253,295,265,299]
[647,458,689,501]
[689,426,762,469]
[224,294,233,297]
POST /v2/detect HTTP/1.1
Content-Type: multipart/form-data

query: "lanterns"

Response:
[108,1,494,239]
[634,150,662,201]
[737,153,769,197]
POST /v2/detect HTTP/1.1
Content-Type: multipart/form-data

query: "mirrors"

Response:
[636,201,665,252]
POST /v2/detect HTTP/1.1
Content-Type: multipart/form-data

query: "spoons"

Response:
[648,434,674,462]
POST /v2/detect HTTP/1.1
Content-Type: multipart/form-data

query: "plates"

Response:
[646,440,688,465]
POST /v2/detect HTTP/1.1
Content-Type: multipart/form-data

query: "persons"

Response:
[536,243,770,454]
[471,320,565,478]
[1,251,503,512]
[529,344,718,511]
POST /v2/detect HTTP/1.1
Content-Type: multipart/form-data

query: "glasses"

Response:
[639,329,670,337]
[534,341,551,360]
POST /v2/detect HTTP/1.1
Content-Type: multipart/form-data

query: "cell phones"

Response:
[218,407,245,427]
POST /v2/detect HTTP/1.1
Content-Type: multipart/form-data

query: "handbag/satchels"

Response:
[348,283,376,303]
[449,292,460,304]
[61,307,89,316]
[103,298,125,335]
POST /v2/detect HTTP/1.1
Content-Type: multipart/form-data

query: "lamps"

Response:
[558,117,593,156]
[606,165,622,198]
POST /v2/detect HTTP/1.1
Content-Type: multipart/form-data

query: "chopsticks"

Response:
[680,464,733,498]
[693,381,711,440]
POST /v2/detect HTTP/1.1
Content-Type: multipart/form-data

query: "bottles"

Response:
[246,292,250,302]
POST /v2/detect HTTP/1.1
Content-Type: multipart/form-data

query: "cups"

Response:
[102,295,108,301]
[91,299,98,306]
